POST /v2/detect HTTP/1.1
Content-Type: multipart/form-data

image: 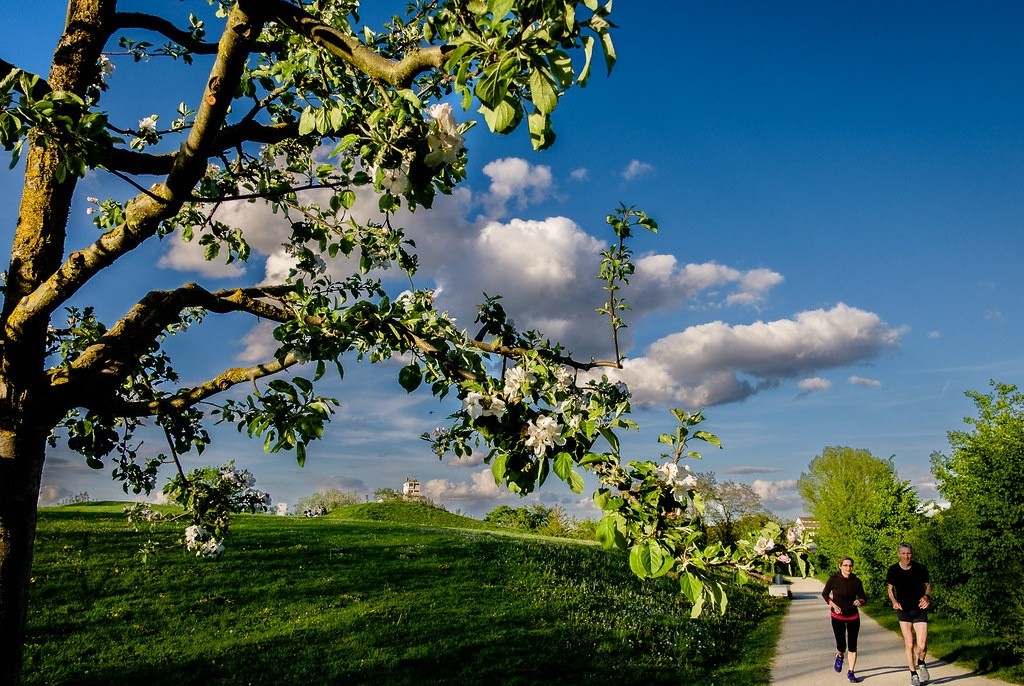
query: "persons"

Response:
[886,541,933,686]
[822,555,868,684]
[303,505,327,519]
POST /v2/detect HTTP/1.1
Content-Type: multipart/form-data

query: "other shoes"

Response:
[847,671,857,683]
[919,665,930,682]
[910,675,920,686]
[834,653,844,673]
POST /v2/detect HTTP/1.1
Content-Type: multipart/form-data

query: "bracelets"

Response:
[924,594,931,600]
